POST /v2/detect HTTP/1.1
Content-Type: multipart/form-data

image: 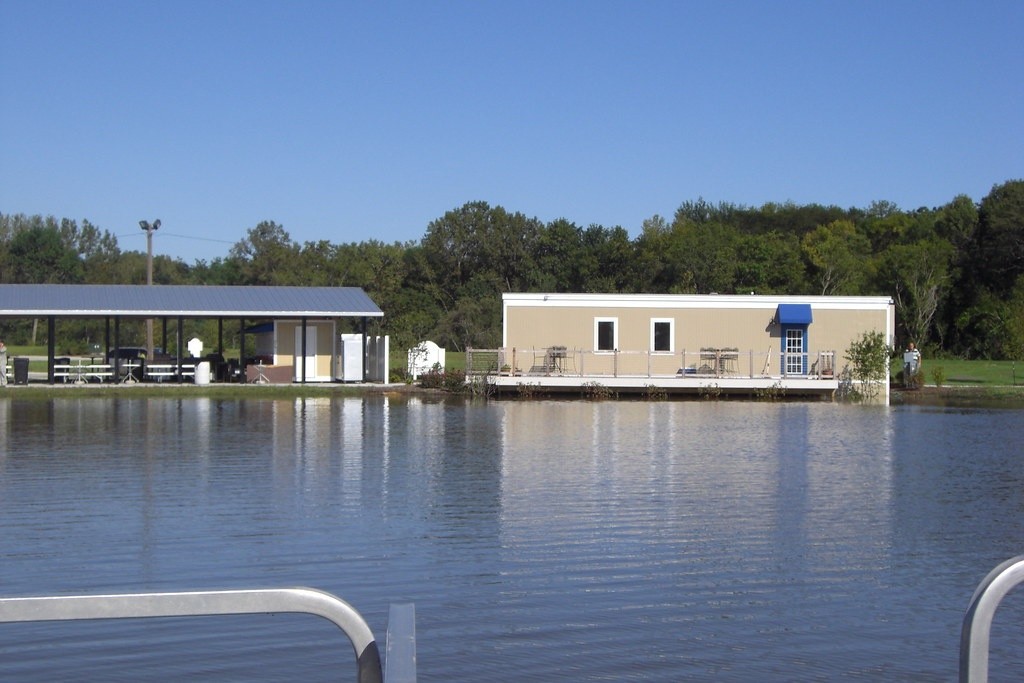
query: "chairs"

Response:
[559,346,579,377]
[530,346,550,377]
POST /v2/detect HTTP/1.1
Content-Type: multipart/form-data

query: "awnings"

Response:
[778,304,812,328]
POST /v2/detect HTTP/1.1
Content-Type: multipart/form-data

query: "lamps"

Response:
[770,317,778,327]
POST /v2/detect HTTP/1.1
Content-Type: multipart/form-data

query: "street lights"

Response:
[139,220,163,380]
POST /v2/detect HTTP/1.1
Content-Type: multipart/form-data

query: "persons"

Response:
[0,338,7,386]
[903,340,922,387]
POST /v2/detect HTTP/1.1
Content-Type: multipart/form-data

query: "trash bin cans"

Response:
[54,358,70,383]
[13,357,29,385]
[194,361,210,384]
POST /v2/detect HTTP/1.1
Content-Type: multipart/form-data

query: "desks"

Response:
[541,346,567,378]
[697,348,741,379]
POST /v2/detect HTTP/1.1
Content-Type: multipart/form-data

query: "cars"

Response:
[101,346,171,376]
